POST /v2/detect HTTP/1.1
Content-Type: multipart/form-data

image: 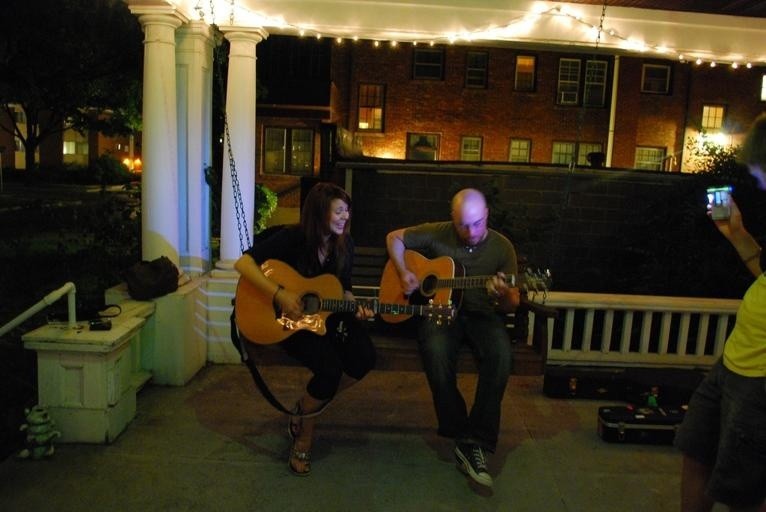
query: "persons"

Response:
[233,180,376,474]
[672,109,765,510]
[385,187,522,487]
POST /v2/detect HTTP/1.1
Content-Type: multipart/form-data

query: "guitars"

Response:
[379,249,556,324]
[234,260,458,345]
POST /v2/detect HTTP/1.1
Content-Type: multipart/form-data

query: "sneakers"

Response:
[455,440,494,489]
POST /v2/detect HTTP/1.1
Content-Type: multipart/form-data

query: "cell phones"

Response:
[706,185,733,222]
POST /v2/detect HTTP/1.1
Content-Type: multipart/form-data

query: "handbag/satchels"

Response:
[50,274,107,322]
[126,257,181,302]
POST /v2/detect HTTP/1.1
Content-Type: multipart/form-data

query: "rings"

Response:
[496,290,498,293]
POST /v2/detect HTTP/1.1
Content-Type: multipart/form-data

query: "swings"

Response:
[209,0,606,376]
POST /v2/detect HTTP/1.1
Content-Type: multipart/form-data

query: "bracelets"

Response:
[742,247,763,267]
[271,283,285,306]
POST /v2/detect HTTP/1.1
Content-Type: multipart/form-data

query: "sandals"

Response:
[289,433,312,476]
[287,402,304,439]
